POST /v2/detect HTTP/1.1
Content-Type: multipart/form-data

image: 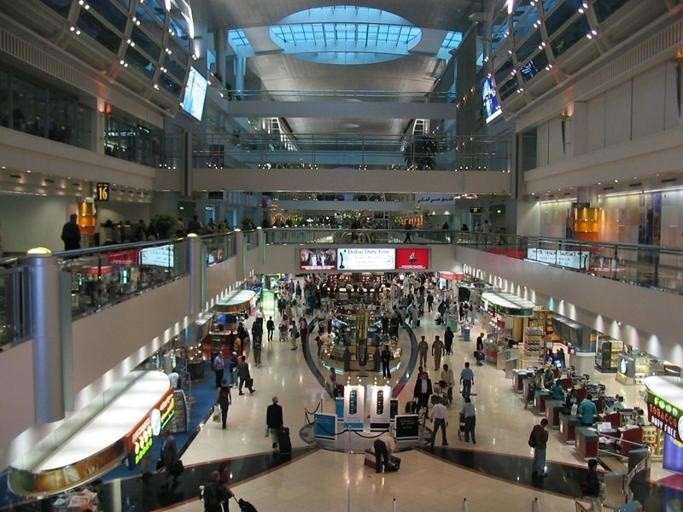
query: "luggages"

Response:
[278,427,292,454]
[238,497,258,512]
[404,400,417,412]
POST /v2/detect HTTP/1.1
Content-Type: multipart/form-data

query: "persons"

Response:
[160,429,179,488]
[459,398,476,443]
[373,435,397,473]
[524,348,603,511]
[60,213,229,307]
[217,462,234,511]
[272,215,336,228]
[203,271,517,419]
[214,379,231,429]
[203,470,228,511]
[335,220,505,248]
[265,396,283,448]
[429,398,448,450]
[306,252,333,266]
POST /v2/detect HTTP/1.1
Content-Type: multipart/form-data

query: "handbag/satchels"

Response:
[528,432,536,448]
[473,351,485,361]
[244,377,254,388]
[169,460,184,478]
